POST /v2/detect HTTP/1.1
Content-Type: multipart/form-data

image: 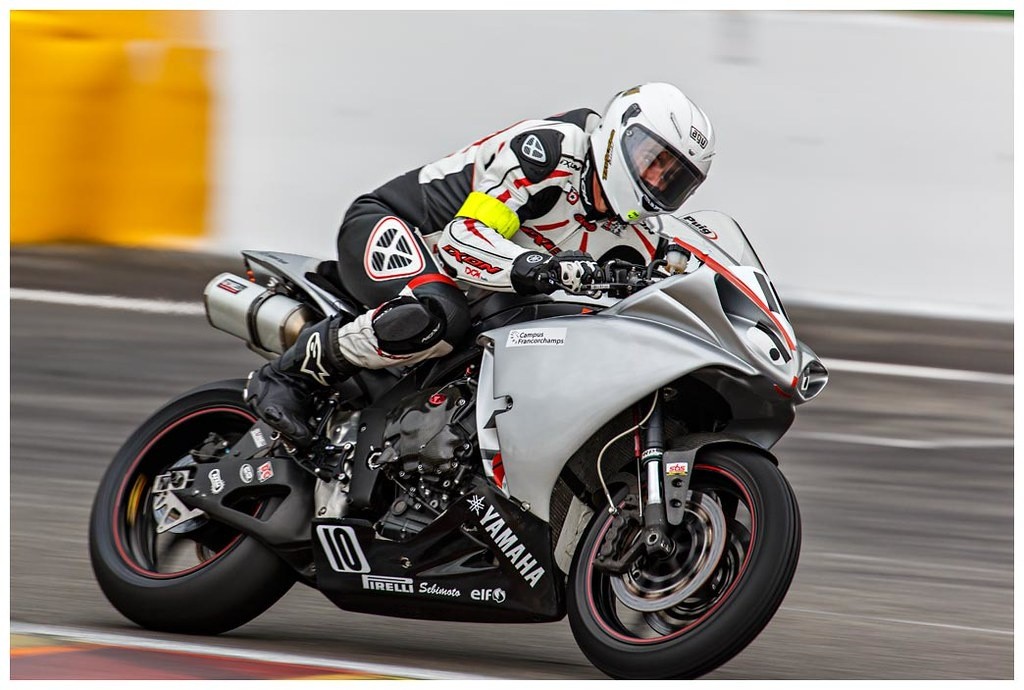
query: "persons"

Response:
[244,82,716,439]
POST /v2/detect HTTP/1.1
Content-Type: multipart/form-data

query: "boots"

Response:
[243,313,362,443]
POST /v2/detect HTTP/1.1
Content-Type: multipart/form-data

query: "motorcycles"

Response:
[86,210,831,680]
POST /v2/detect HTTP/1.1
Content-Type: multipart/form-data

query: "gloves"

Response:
[511,246,605,298]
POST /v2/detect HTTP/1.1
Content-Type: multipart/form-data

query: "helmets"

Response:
[592,80,715,223]
